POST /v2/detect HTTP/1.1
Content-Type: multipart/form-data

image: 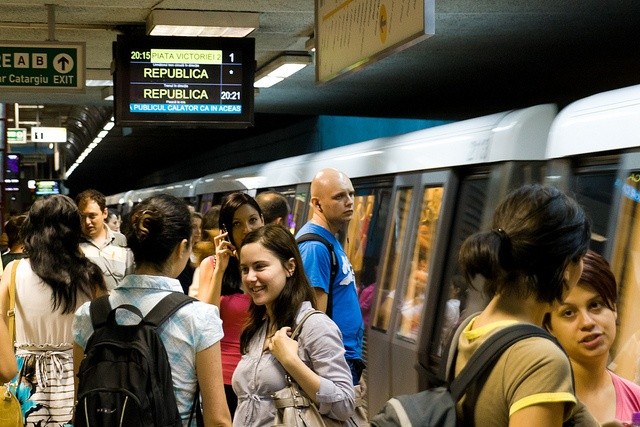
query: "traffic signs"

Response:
[1,41,86,93]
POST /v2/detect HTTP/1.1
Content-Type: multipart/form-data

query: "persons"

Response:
[229,222,367,427]
[72,192,233,427]
[254,190,291,232]
[186,191,266,427]
[435,277,466,359]
[539,248,639,427]
[104,207,132,238]
[292,168,368,402]
[368,256,428,342]
[344,196,367,329]
[176,202,222,296]
[1,204,29,271]
[0,193,111,426]
[73,188,137,297]
[435,183,604,427]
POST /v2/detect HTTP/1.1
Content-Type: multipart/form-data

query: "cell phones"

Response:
[218,222,234,252]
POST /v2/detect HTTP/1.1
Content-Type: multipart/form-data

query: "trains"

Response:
[101,84,640,424]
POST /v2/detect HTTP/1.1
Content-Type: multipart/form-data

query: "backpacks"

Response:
[74,291,204,427]
[372,310,548,427]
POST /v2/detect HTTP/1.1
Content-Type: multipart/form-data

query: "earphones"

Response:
[315,201,321,209]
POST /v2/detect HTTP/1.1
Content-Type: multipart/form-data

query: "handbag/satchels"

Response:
[0,386,24,427]
[269,382,371,427]
